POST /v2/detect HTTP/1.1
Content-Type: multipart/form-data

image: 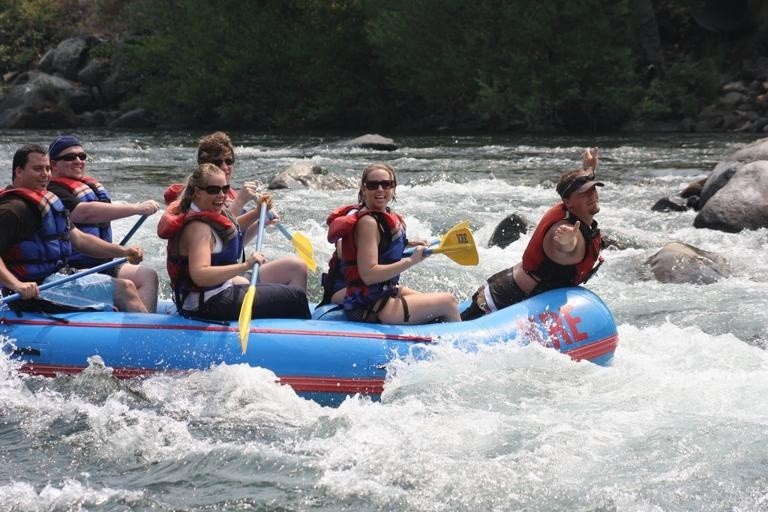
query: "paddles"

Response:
[406,219,471,248]
[237,200,267,356]
[252,195,318,273]
[402,227,480,266]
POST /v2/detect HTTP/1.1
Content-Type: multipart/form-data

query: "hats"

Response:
[556,169,605,199]
[50,136,82,161]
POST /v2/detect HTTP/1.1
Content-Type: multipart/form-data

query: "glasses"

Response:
[198,157,234,167]
[51,152,87,162]
[562,173,595,197]
[362,180,395,190]
[196,184,230,194]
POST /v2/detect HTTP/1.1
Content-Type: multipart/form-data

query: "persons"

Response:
[182,133,308,298]
[460,146,605,321]
[167,163,312,320]
[341,164,462,325]
[0,143,149,318]
[322,167,431,307]
[47,135,160,314]
